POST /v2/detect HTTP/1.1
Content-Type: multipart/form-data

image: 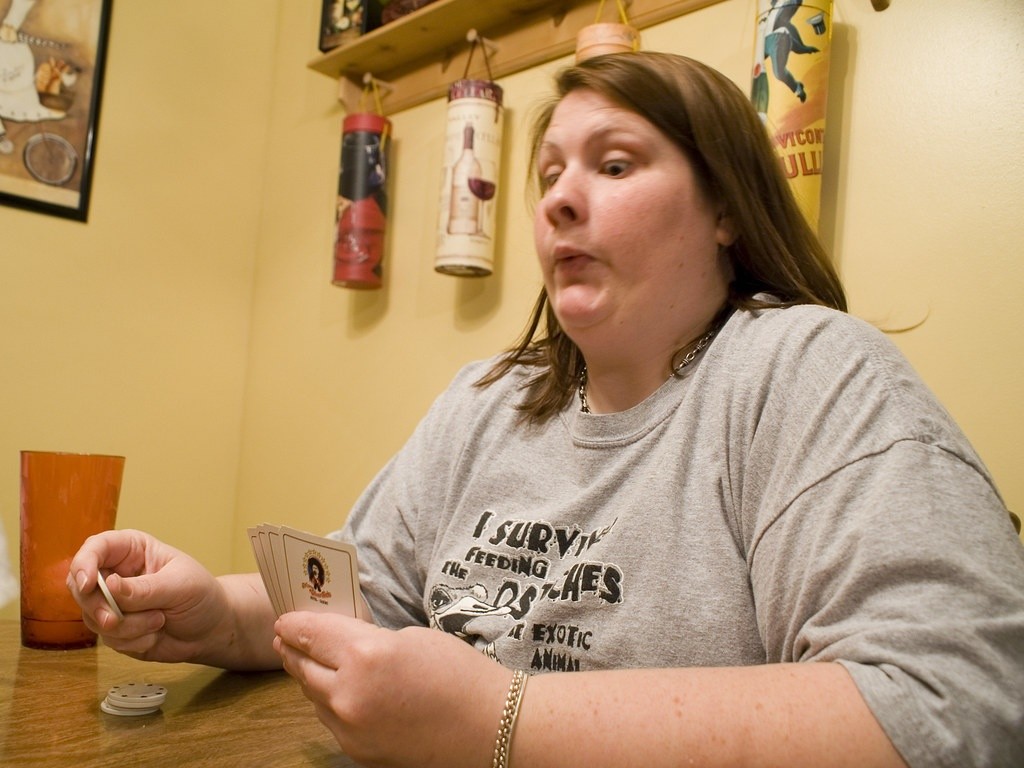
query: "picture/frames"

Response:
[0,0,113,222]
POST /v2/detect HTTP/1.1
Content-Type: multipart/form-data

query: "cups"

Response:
[19,450,126,652]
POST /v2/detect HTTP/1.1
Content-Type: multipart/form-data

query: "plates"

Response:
[23,131,78,185]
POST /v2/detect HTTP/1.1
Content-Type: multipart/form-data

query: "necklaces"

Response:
[579,319,717,412]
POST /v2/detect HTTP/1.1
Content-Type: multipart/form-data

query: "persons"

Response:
[66,51,1024,768]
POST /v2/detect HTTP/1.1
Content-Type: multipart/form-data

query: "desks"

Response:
[0,619,369,768]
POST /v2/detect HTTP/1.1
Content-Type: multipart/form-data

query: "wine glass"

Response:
[468,159,496,240]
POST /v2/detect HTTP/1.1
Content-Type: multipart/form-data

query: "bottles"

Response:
[447,122,482,235]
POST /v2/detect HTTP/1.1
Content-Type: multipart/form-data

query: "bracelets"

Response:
[492,670,527,768]
